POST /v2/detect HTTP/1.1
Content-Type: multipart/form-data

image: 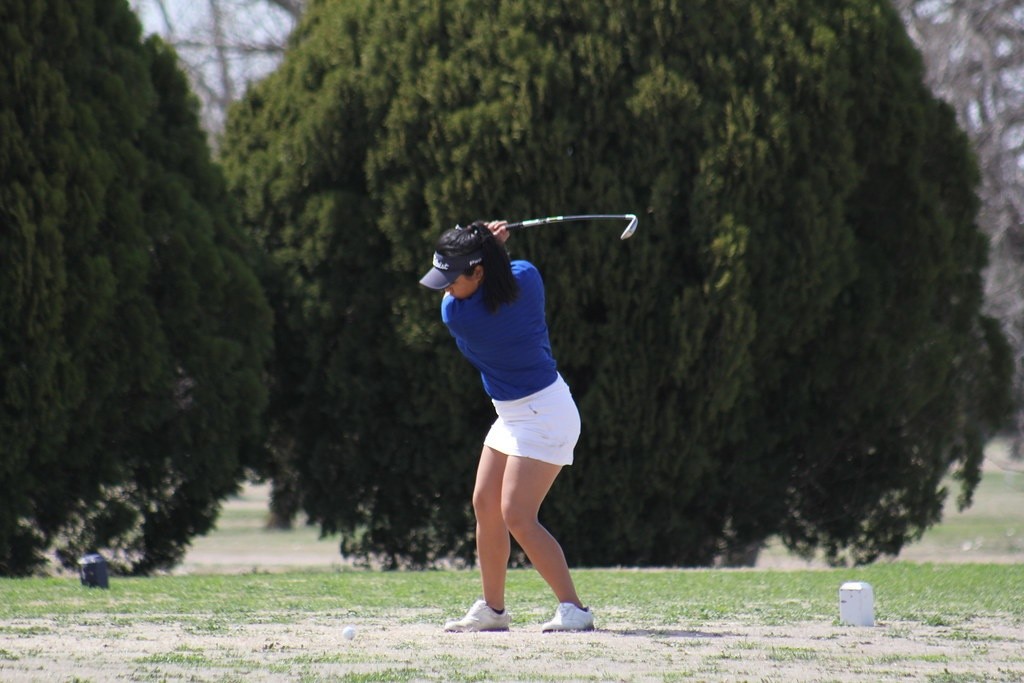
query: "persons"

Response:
[417,218,594,632]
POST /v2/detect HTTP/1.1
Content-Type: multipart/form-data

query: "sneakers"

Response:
[445,599,509,632]
[542,602,594,632]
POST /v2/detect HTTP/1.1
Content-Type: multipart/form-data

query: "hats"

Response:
[419,251,484,290]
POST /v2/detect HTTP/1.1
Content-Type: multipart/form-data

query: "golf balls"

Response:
[342,627,356,639]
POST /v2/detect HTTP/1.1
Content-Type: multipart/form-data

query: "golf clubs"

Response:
[501,214,639,241]
